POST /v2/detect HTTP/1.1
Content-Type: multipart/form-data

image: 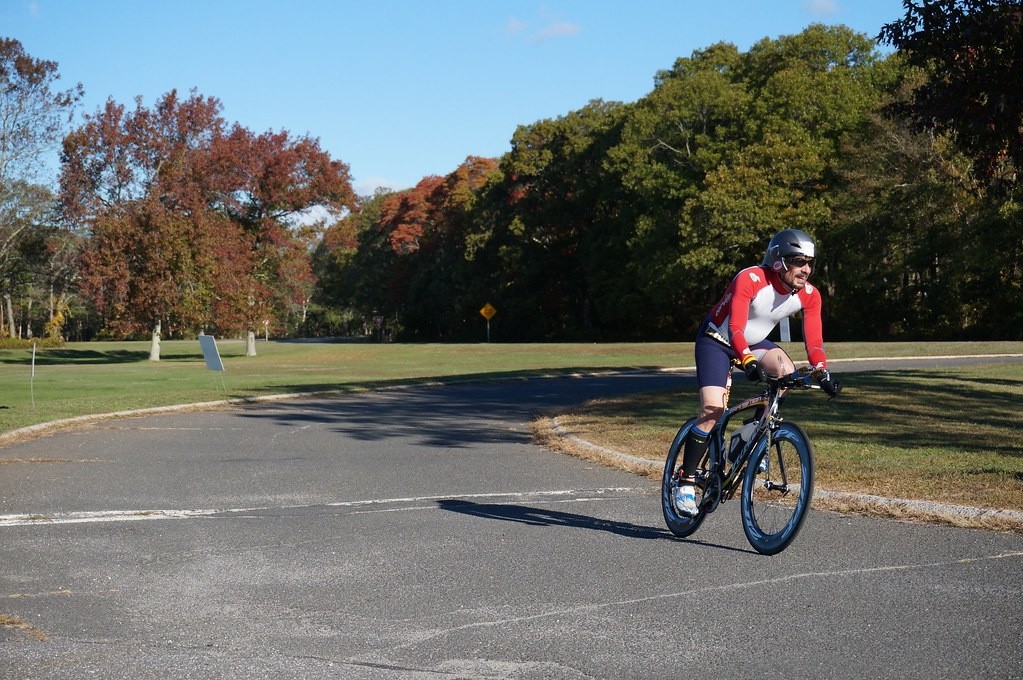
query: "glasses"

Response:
[783,258,814,267]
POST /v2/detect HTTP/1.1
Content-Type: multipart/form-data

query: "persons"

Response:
[670,228,831,518]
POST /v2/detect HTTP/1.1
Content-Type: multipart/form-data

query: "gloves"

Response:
[744,360,767,383]
[817,373,843,397]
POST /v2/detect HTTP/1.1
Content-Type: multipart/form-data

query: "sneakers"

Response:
[670,465,699,519]
[747,441,765,471]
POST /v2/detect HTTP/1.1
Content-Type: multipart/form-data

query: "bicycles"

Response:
[662,357,837,556]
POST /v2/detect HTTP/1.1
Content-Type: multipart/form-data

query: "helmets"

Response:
[762,229,815,272]
